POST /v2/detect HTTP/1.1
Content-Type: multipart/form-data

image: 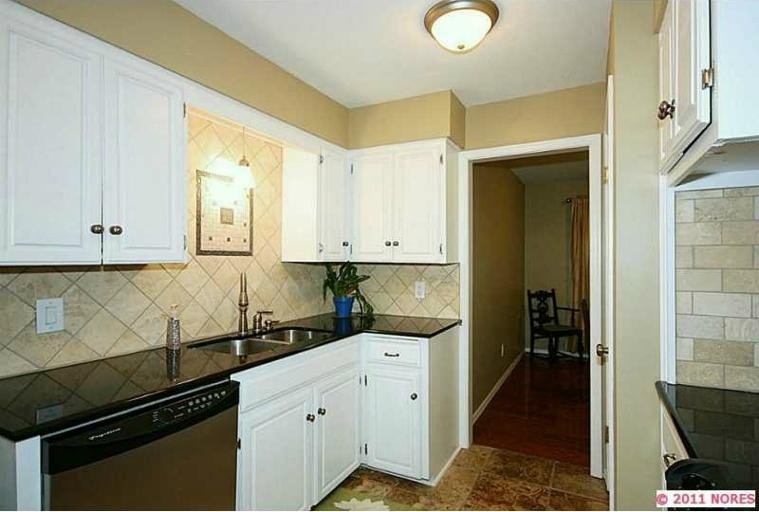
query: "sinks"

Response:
[250,325,334,344]
[187,337,287,356]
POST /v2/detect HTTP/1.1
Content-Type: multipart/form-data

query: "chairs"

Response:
[526,287,584,369]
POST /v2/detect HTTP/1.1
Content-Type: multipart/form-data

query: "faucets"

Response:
[237,272,248,333]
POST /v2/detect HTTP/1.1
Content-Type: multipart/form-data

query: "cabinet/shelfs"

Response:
[277,146,342,265]
[649,0,757,190]
[364,333,460,488]
[344,137,459,264]
[226,338,362,511]
[0,1,189,267]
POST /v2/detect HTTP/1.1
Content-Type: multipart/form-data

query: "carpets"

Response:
[315,432,610,512]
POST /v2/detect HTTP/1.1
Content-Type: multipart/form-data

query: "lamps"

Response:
[424,0,499,55]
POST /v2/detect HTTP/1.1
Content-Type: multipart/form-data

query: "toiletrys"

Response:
[166,305,180,351]
[166,351,181,385]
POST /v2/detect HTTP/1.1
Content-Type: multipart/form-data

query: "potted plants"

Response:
[319,261,374,321]
